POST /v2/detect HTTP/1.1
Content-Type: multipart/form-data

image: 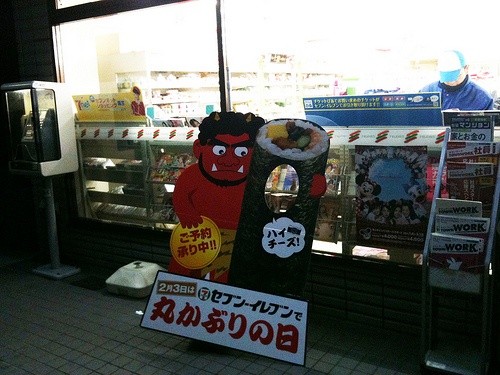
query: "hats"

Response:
[134,87,140,94]
[438,50,466,82]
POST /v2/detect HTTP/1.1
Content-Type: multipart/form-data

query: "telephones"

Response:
[21,108,58,146]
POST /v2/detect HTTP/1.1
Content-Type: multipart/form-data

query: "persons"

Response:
[315,205,339,241]
[324,162,335,190]
[158,118,202,127]
[447,179,460,193]
[355,145,427,224]
[125,87,146,116]
[418,50,494,110]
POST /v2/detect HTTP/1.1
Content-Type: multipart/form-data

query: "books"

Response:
[264,164,300,212]
[88,202,180,224]
[427,113,500,295]
[147,150,197,184]
[83,157,144,170]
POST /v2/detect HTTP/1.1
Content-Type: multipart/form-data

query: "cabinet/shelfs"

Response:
[75,70,500,375]
